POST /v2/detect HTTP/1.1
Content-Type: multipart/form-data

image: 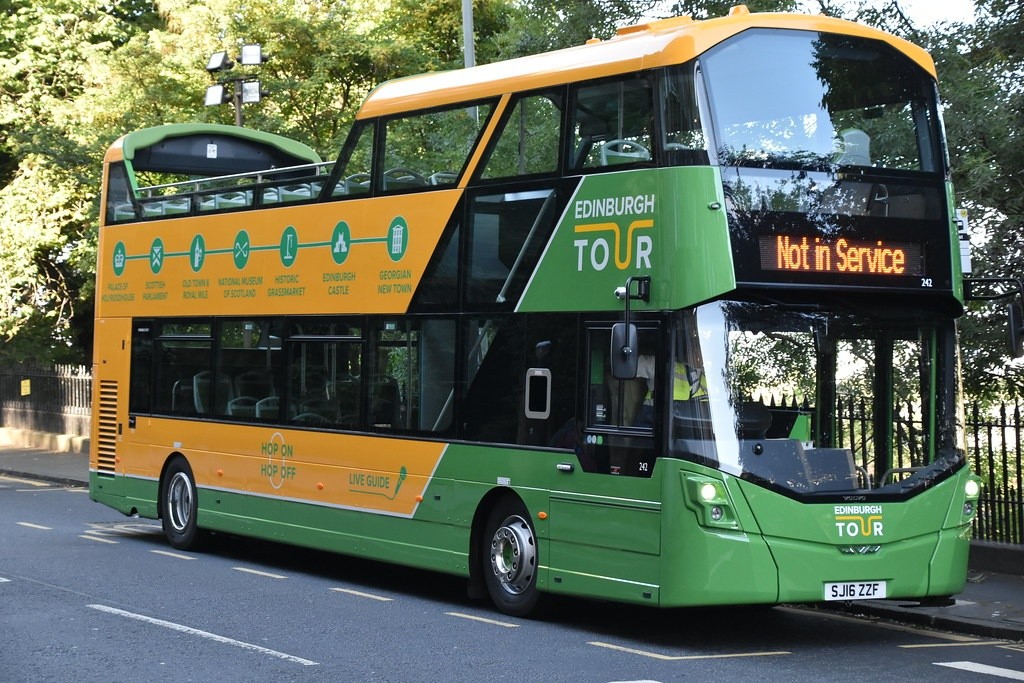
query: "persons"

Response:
[634,336,708,428]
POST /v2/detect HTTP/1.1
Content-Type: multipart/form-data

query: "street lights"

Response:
[205,44,270,126]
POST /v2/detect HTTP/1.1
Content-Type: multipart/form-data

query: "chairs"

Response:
[596,141,699,169]
[108,164,459,222]
[149,367,416,427]
[835,126,873,165]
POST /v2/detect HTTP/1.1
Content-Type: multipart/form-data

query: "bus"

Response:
[88,4,1024,620]
